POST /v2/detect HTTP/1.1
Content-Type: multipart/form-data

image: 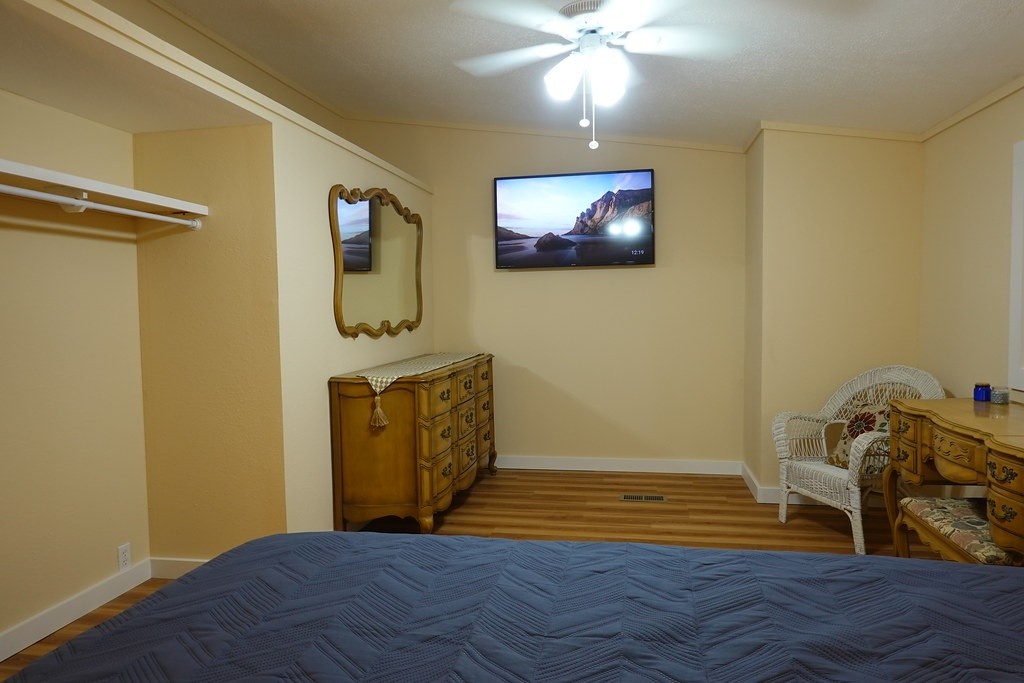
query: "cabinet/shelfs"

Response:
[327,351,498,535]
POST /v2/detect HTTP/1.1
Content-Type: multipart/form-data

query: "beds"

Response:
[0,530,1024,683]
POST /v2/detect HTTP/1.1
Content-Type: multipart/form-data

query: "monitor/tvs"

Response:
[337,196,372,271]
[494,169,655,269]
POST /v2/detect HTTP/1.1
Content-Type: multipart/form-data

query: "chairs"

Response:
[771,365,946,556]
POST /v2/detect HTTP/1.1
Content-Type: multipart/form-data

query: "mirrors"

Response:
[330,184,424,339]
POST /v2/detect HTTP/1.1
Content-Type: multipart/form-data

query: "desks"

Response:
[882,398,1024,565]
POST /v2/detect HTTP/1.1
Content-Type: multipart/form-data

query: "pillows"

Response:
[825,401,892,476]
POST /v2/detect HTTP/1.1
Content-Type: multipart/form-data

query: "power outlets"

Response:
[118,542,132,572]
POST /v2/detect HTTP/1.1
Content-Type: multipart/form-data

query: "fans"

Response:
[448,1,745,109]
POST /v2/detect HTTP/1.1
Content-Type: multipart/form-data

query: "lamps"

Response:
[547,35,637,152]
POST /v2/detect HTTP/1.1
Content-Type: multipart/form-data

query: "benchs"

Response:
[893,496,1023,566]
